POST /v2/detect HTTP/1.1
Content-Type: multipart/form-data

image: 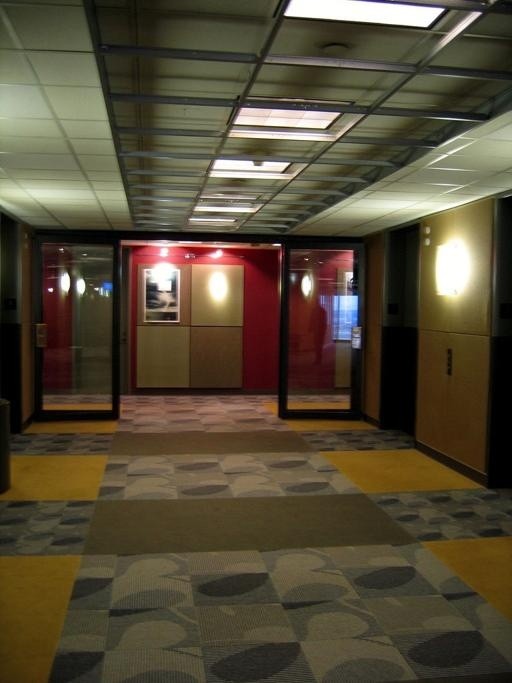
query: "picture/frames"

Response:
[142,268,181,323]
